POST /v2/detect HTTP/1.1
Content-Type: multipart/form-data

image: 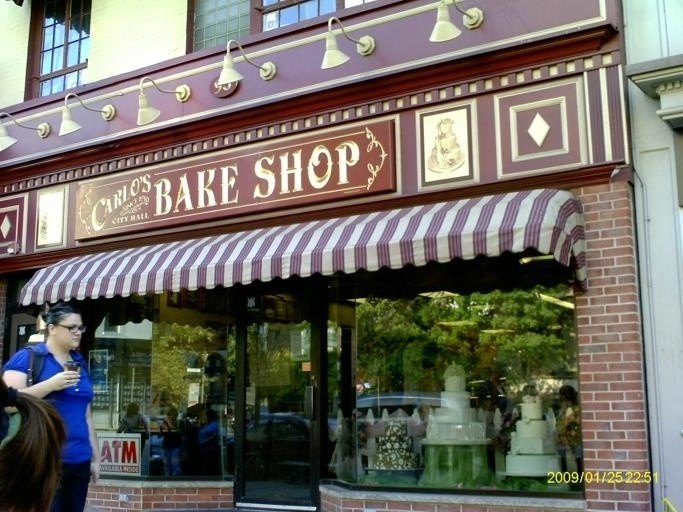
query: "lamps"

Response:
[319,15,377,72]
[58,91,119,138]
[0,110,51,153]
[216,39,278,88]
[135,76,194,127]
[428,1,485,46]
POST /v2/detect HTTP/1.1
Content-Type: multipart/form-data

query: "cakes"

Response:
[373,421,420,470]
[430,119,462,169]
[506,395,561,473]
[425,364,486,440]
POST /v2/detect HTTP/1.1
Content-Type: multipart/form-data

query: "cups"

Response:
[62,358,77,371]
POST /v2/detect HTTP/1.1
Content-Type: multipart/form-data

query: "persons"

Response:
[0,302,101,512]
[0,379,67,511]
[114,401,148,454]
[195,408,220,475]
[553,384,582,488]
[157,408,184,477]
[66,380,70,384]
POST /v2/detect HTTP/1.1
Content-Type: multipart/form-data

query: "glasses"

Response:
[54,324,87,333]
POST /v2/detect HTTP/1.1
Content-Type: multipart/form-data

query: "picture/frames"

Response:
[30,182,70,254]
[412,97,482,193]
